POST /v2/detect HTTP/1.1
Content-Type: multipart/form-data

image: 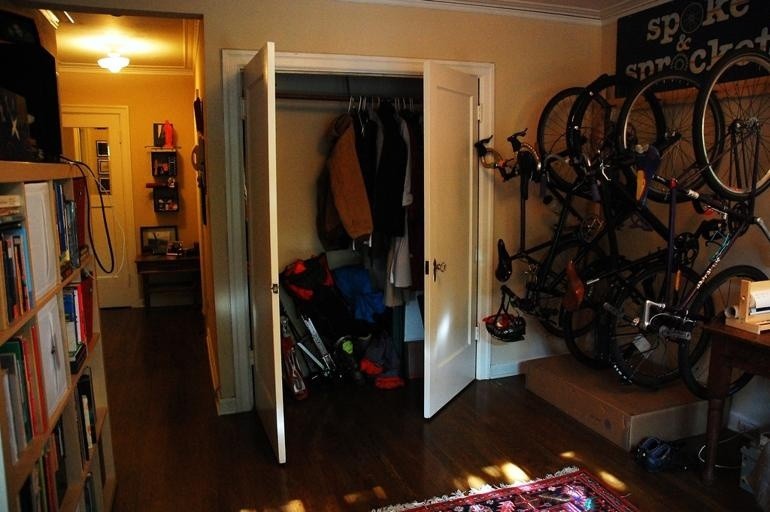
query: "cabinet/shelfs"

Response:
[150,151,178,213]
[0,158,119,512]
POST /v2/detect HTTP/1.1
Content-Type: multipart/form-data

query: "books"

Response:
[0,177,110,512]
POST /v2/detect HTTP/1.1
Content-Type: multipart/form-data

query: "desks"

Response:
[136,254,203,315]
[697,321,770,494]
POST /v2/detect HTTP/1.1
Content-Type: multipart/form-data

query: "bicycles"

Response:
[474,46,770,398]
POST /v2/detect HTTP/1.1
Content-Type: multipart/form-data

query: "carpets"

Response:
[367,465,643,512]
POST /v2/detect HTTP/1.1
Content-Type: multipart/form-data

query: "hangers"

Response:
[346,90,415,135]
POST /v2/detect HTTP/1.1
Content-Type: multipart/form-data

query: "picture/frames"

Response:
[139,226,178,256]
[95,140,111,193]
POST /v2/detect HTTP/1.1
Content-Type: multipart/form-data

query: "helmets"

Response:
[483,314,526,342]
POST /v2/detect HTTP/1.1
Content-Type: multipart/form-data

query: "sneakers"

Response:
[635,436,660,461]
[646,445,675,473]
